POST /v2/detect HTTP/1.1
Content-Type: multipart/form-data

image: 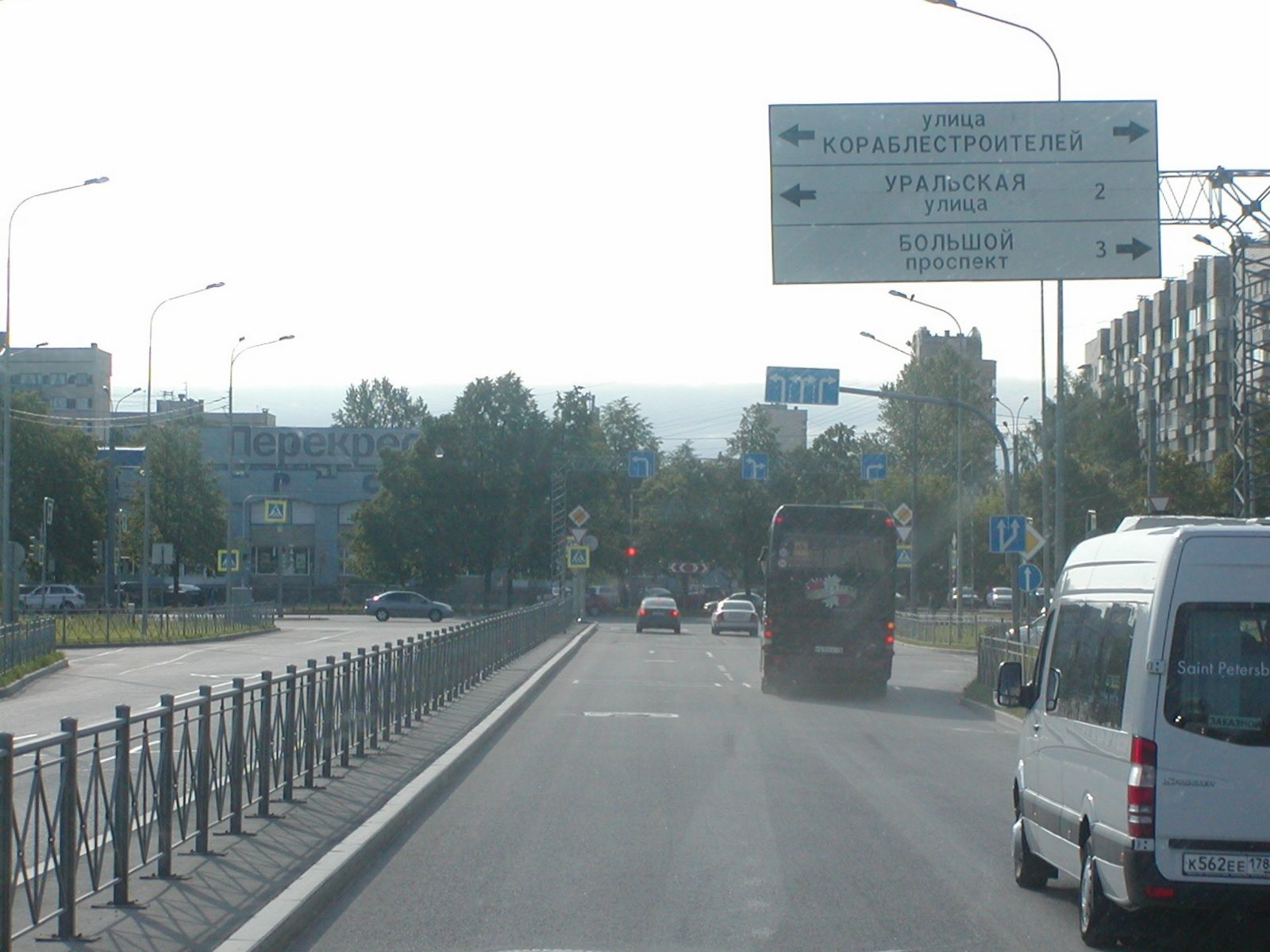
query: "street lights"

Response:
[141,282,227,631]
[858,331,919,613]
[1100,354,1155,514]
[0,175,109,631]
[925,0,1068,589]
[228,333,295,622]
[101,385,141,609]
[989,394,1029,657]
[889,290,965,642]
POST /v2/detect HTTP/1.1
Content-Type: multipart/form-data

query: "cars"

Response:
[690,585,765,637]
[1007,611,1049,646]
[584,585,675,615]
[364,590,455,623]
[19,575,203,611]
[948,585,1014,609]
[636,597,681,634]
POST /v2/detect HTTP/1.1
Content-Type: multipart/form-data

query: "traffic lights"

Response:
[626,547,636,557]
[92,540,102,561]
[26,536,38,562]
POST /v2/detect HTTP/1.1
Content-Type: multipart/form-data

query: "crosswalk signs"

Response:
[217,550,239,571]
[262,499,288,524]
[898,544,912,568]
[566,546,590,569]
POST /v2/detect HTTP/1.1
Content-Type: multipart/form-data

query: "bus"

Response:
[759,503,903,693]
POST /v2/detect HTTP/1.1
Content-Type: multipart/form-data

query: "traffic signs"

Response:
[768,96,1162,285]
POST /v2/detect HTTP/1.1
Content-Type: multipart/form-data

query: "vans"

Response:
[997,517,1268,952]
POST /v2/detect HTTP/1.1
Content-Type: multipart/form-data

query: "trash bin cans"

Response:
[232,587,252,622]
